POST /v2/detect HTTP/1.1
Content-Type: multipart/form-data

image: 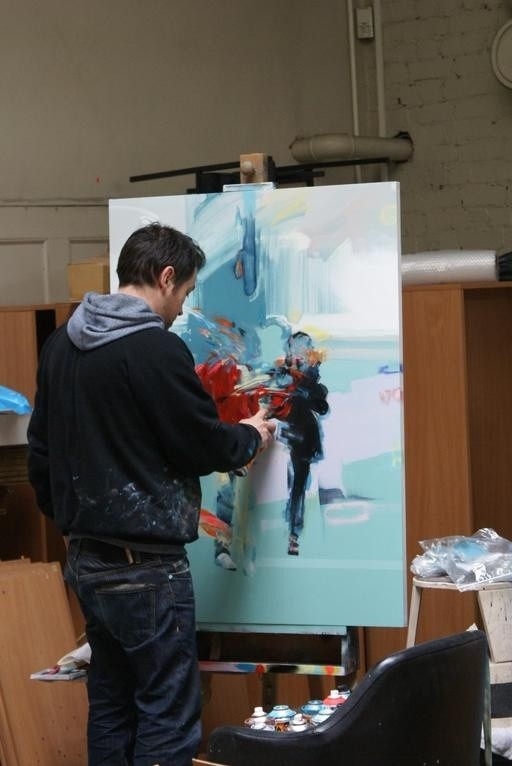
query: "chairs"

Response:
[207,627,489,765]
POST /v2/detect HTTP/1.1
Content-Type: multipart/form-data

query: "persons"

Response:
[27,223,276,766]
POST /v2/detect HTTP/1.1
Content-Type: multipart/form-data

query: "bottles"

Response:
[243,684,353,733]
